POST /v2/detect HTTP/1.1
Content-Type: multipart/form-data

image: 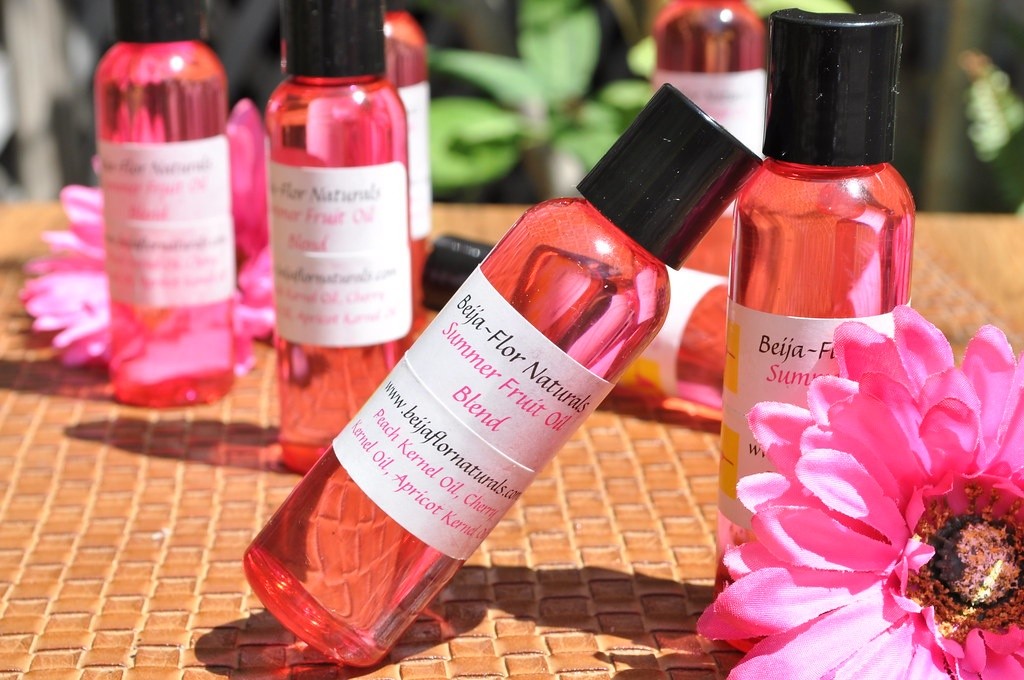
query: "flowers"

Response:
[18,97,274,382]
[695,300,1023,680]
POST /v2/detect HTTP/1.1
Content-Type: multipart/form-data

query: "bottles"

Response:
[263,0,412,475]
[385,0,431,331]
[713,7,914,655]
[423,234,730,422]
[93,0,233,406]
[245,84,762,668]
[653,0,766,277]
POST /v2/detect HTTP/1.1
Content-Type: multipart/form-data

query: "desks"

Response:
[0,198,1023,680]
